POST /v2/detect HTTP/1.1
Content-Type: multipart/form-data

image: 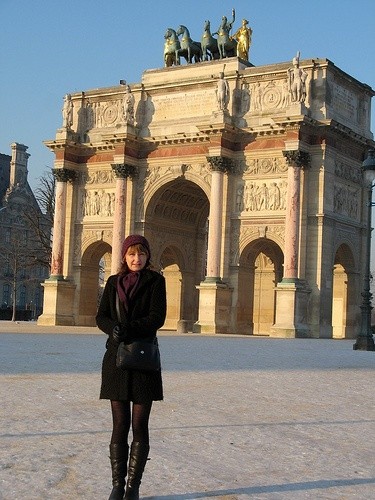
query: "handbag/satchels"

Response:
[116,339,161,372]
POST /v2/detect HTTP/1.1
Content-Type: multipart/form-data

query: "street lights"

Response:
[352,148,375,352]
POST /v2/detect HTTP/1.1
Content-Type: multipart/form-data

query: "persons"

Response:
[86,102,104,129]
[236,182,287,212]
[61,94,74,133]
[95,234,166,500]
[121,84,135,126]
[80,190,116,217]
[287,57,307,103]
[278,79,289,109]
[241,81,262,113]
[214,71,229,112]
[115,100,124,127]
[229,19,252,63]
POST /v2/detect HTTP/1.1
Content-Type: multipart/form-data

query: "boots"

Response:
[109,442,150,500]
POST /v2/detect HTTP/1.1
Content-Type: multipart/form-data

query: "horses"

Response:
[162,17,237,67]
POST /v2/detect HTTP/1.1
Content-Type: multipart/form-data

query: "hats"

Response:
[121,234,151,260]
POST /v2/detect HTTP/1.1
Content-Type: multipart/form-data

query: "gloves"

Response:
[113,323,127,343]
